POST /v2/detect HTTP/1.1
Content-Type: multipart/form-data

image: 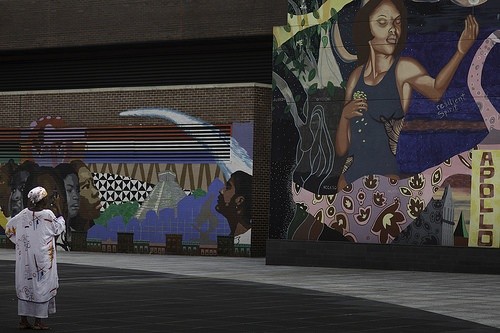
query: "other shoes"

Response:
[34,325,49,330]
[16,322,34,329]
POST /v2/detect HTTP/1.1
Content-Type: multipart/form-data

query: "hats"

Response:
[27,185,47,206]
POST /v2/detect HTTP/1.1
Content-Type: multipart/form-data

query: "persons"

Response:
[5,186,67,330]
